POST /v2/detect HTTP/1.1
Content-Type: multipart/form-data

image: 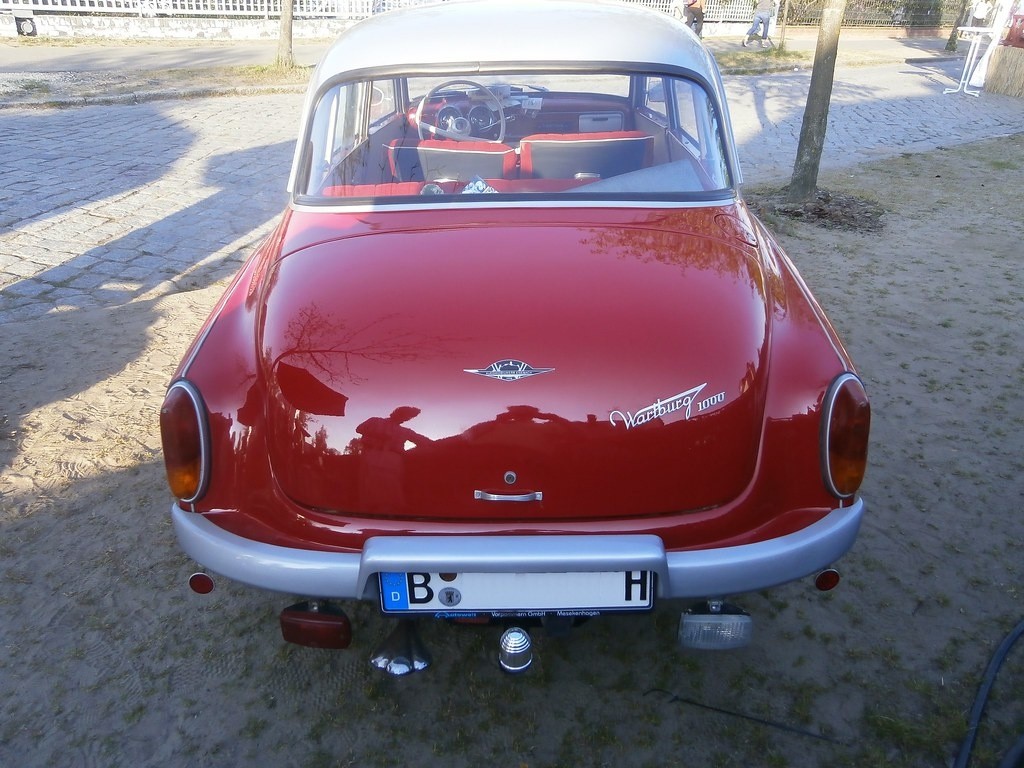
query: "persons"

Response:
[968,0,994,36]
[686,0,706,35]
[671,0,684,20]
[743,0,771,48]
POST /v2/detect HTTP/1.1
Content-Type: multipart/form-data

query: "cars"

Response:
[160,0,872,676]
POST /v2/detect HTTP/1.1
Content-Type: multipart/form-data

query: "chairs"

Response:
[520,130,657,182]
[387,137,514,187]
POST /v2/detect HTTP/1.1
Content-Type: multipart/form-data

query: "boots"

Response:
[742,34,749,47]
[761,39,767,48]
